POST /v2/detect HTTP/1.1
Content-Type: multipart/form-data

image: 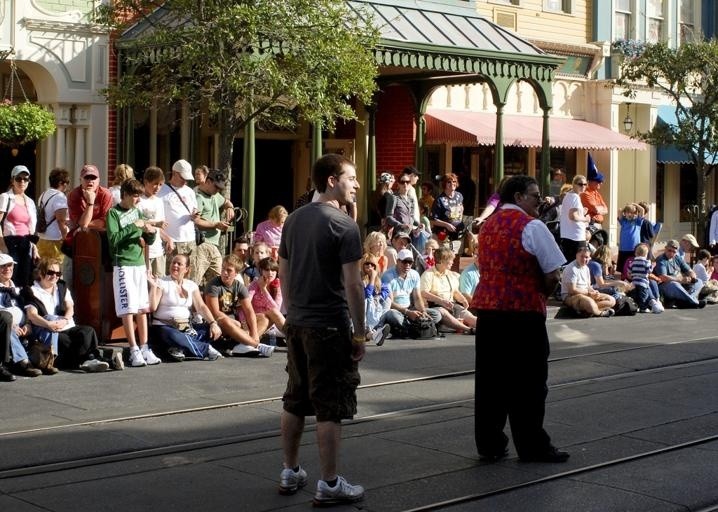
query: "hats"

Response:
[172,159,195,180]
[683,233,699,248]
[12,166,30,177]
[396,249,413,260]
[80,165,99,178]
[208,169,226,189]
[404,167,422,175]
[0,254,17,266]
[393,232,412,241]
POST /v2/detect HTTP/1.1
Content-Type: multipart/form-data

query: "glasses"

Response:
[46,270,61,277]
[365,261,375,267]
[400,180,409,185]
[14,176,30,183]
[402,260,413,265]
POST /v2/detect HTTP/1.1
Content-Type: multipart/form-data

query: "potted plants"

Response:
[0,97,57,157]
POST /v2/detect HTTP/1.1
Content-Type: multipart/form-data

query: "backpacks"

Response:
[36,191,60,232]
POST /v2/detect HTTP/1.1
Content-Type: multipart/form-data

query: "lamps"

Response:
[623,102,632,133]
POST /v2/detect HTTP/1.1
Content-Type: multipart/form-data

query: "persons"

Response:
[535,175,718,316]
[467,176,570,464]
[361,168,512,346]
[0,160,289,382]
[276,155,367,506]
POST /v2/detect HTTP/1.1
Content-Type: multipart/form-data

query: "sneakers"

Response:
[19,364,42,377]
[0,366,16,381]
[256,343,276,357]
[168,347,185,362]
[80,359,109,371]
[315,475,364,503]
[43,367,57,375]
[601,309,615,317]
[637,302,664,312]
[699,301,706,308]
[538,449,569,462]
[113,353,125,370]
[130,350,162,367]
[207,344,221,360]
[280,465,308,494]
[372,324,392,345]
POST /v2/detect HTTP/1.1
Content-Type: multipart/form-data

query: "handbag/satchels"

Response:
[448,222,466,239]
[407,317,436,339]
[28,339,55,368]
[615,296,637,316]
[195,228,202,246]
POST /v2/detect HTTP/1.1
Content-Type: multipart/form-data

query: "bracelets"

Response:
[353,336,366,343]
